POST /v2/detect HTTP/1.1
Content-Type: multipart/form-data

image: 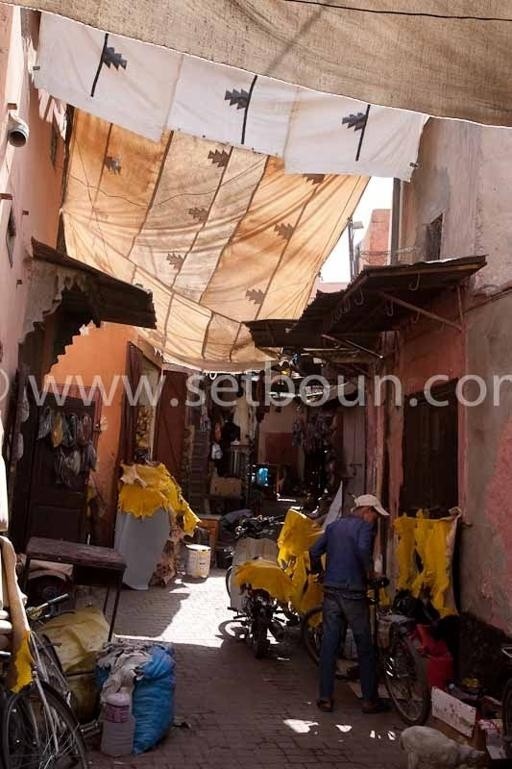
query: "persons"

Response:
[220,414,241,469]
[306,490,398,716]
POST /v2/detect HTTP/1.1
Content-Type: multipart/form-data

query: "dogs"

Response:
[399,724,486,769]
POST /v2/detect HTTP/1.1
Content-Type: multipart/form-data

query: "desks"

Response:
[22,537,129,645]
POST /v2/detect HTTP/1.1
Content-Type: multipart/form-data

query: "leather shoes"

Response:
[316,699,332,712]
[359,697,389,715]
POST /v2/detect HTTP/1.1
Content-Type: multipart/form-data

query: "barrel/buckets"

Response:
[100,687,138,757]
[426,656,452,694]
[184,542,213,579]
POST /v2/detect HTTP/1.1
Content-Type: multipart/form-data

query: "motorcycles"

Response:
[222,511,286,665]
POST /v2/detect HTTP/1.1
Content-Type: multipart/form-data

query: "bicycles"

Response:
[280,504,439,726]
[0,594,95,768]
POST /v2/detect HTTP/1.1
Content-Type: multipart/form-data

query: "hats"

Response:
[350,494,390,518]
